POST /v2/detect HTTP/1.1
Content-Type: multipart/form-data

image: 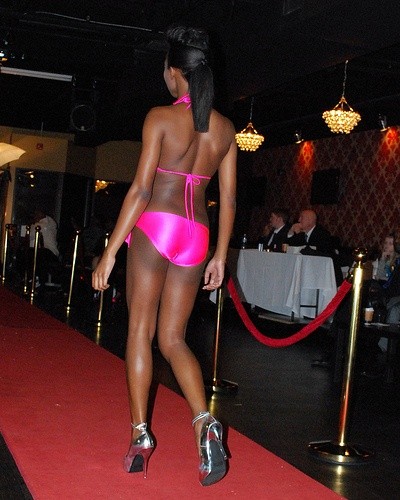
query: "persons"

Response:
[260,209,400,352]
[23,205,61,287]
[93,29,237,487]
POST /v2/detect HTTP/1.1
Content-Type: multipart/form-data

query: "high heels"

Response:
[122,422,155,479]
[192,410,227,487]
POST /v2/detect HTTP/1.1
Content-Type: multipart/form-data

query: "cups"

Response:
[282,242,290,253]
[363,307,374,323]
[259,244,263,251]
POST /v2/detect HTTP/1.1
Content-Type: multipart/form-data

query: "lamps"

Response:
[322,58,361,134]
[293,130,303,145]
[235,95,264,152]
[378,114,390,132]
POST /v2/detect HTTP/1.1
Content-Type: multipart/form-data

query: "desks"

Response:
[333,320,399,384]
[235,248,336,322]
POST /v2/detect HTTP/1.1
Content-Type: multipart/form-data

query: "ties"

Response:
[305,234,308,240]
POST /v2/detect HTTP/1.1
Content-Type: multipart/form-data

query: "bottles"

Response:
[241,234,248,250]
